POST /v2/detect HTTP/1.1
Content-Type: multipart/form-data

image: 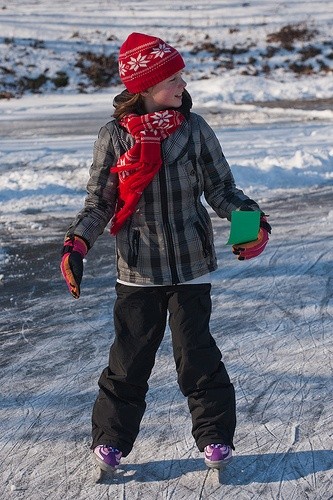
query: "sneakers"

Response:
[203,443,233,468]
[93,445,123,474]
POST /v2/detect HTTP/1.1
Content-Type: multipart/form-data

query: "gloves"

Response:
[60,236,88,300]
[232,215,272,259]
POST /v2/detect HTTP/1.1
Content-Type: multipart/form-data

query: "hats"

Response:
[117,33,185,95]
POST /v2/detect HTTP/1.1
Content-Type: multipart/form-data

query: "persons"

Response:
[59,31,271,473]
